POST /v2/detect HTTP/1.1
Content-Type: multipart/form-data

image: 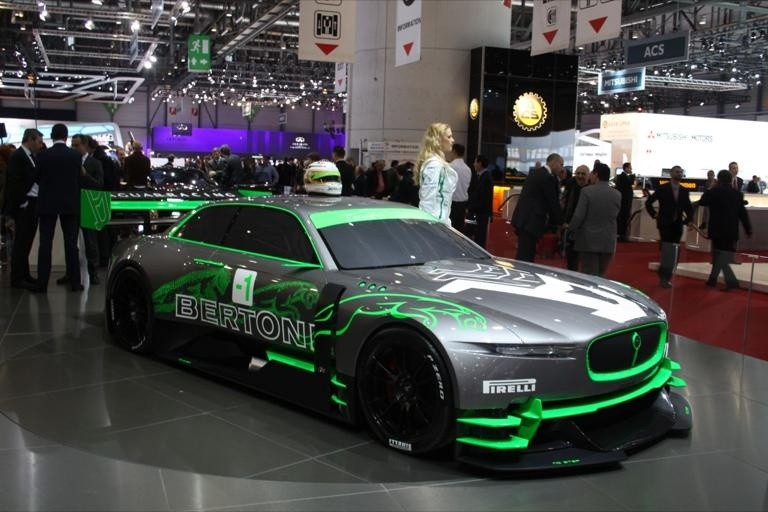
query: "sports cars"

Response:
[79,189,691,473]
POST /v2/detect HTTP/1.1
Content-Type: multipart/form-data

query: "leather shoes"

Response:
[719,281,738,291]
[89,271,99,285]
[661,277,671,288]
[11,276,47,294]
[704,279,715,287]
[56,275,65,285]
[72,284,83,291]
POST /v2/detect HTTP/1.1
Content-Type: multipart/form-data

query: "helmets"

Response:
[303,159,342,195]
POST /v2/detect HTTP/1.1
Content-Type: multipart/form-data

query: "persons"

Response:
[2,123,151,293]
[413,124,458,227]
[512,153,635,277]
[747,176,763,194]
[645,165,692,290]
[693,162,753,293]
[166,144,321,195]
[469,155,493,249]
[332,145,420,207]
[449,144,472,232]
